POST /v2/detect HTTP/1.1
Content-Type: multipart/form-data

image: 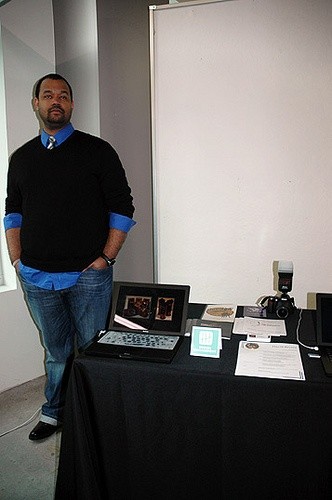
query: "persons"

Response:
[4,74,137,441]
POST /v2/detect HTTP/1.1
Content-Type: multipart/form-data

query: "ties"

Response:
[47,135,56,150]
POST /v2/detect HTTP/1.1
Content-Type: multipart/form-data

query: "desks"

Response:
[53,302,332,500]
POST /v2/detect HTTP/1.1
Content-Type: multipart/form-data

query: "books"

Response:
[184,318,232,340]
[201,304,237,323]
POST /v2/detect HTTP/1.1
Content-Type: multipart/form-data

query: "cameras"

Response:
[266,260,295,319]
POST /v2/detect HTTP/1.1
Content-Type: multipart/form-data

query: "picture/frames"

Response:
[108,281,191,336]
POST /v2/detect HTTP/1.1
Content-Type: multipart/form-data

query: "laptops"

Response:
[84,281,190,364]
[316,293,332,376]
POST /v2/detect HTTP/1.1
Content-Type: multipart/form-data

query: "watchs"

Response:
[100,253,117,267]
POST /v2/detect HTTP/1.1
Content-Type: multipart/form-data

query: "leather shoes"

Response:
[28,421,57,440]
